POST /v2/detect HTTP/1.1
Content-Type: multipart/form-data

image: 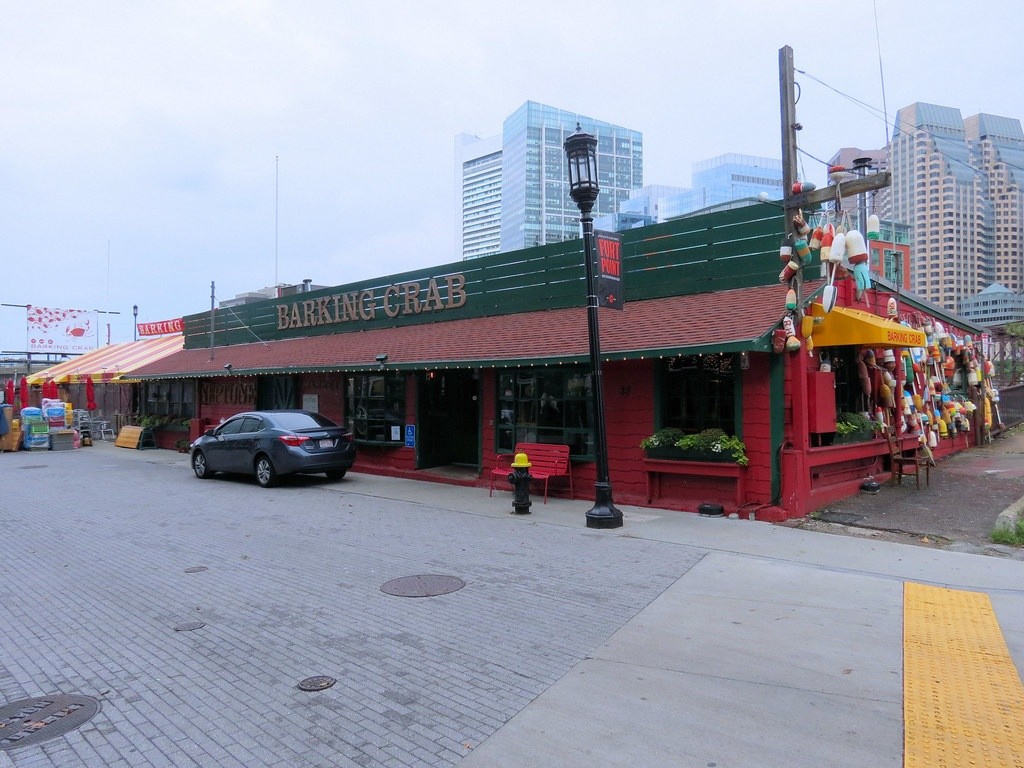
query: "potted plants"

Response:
[175,439,191,453]
[832,411,882,444]
[640,426,750,467]
[140,414,191,432]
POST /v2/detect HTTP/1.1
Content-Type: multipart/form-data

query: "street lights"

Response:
[561,121,625,530]
[132,304,138,342]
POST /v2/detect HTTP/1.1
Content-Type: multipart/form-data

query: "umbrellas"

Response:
[3,372,98,437]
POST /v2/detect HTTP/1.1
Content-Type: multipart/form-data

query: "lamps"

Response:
[376,354,388,364]
[224,364,232,372]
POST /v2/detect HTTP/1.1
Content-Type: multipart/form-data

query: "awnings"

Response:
[809,299,928,349]
[27,330,184,384]
[119,277,832,380]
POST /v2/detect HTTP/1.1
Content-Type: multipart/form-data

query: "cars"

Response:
[189,408,358,489]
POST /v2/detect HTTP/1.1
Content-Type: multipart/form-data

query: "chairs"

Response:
[884,426,930,490]
[74,409,114,447]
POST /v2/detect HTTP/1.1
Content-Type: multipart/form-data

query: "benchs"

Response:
[489,443,573,504]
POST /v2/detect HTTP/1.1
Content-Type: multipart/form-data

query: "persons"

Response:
[537,385,561,436]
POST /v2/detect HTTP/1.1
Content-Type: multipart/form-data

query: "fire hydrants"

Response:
[508,452,532,515]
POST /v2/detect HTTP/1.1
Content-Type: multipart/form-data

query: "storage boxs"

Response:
[21,399,76,451]
